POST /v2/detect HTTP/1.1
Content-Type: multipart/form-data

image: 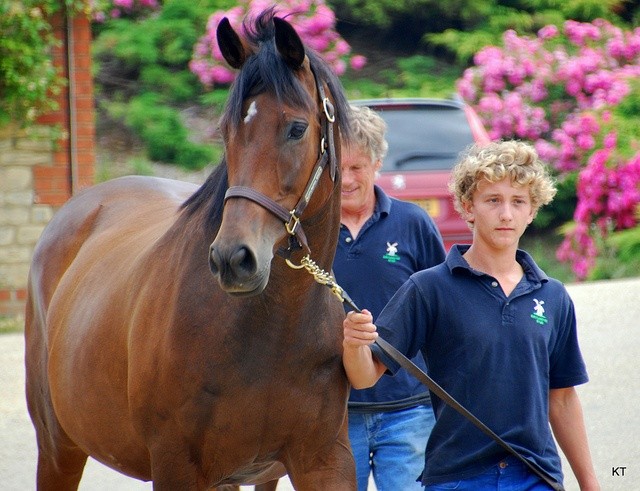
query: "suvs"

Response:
[347,98,493,257]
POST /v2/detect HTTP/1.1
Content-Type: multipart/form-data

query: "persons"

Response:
[342,138,600,491]
[332,106,447,491]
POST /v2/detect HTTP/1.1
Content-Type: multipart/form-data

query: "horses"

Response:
[22,1,358,490]
